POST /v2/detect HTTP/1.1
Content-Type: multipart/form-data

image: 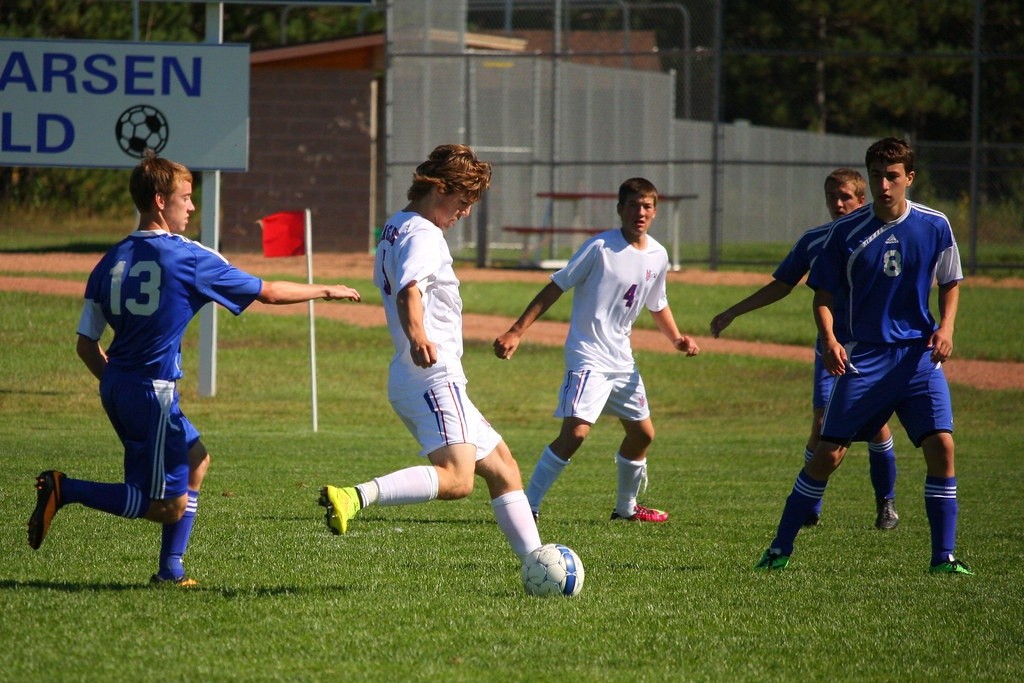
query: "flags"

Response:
[258,207,308,259]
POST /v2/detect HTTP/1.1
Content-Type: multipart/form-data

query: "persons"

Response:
[708,162,902,532]
[490,174,700,527]
[25,148,361,593]
[751,133,984,580]
[316,142,549,571]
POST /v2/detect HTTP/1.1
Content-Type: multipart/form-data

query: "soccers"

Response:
[520,544,585,598]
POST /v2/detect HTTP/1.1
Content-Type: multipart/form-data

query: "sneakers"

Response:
[928,553,977,577]
[150,574,198,586]
[874,495,899,531]
[753,548,790,572]
[26,469,66,550]
[610,504,669,523]
[315,484,352,536]
[803,513,821,529]
